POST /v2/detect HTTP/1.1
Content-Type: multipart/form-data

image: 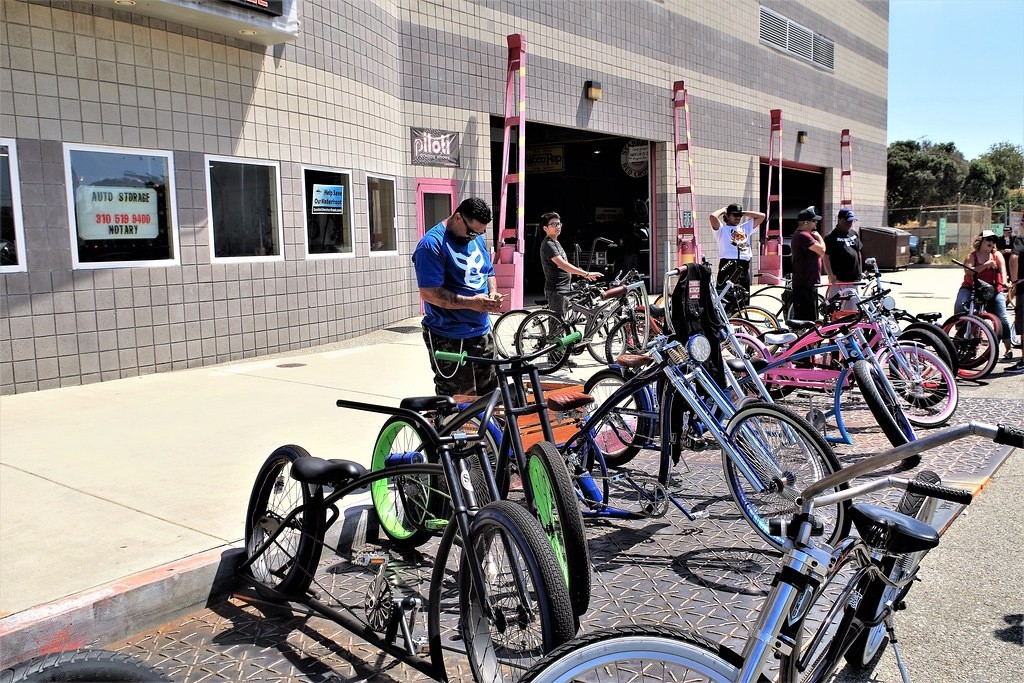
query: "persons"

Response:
[539,211,605,368]
[954,212,1024,374]
[709,203,766,318]
[411,196,504,396]
[790,209,826,321]
[823,207,864,312]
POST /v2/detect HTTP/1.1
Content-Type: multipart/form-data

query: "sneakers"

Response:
[1005,359,1024,374]
[547,353,577,368]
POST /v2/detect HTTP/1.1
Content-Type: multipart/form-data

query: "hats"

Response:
[838,208,858,222]
[797,210,822,222]
[979,230,999,243]
[727,203,745,214]
[1003,226,1012,233]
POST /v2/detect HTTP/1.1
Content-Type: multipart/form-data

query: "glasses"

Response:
[844,220,853,224]
[812,219,816,223]
[547,222,563,228]
[459,212,486,237]
[986,236,997,244]
[731,213,744,217]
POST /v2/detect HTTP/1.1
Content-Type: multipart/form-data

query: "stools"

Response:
[524,223,542,262]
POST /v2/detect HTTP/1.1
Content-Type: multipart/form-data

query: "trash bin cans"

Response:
[860,226,912,271]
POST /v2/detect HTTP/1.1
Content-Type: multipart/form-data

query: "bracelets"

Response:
[585,272,590,278]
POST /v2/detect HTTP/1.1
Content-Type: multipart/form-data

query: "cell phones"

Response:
[496,294,509,301]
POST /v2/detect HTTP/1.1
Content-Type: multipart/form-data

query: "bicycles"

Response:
[233,386,577,683]
[370,330,591,615]
[433,236,1013,553]
[518,418,1024,683]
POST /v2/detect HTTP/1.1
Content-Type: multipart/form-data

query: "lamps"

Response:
[584,80,602,100]
[797,131,807,143]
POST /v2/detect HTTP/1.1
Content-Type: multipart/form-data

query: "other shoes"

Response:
[1001,351,1015,362]
